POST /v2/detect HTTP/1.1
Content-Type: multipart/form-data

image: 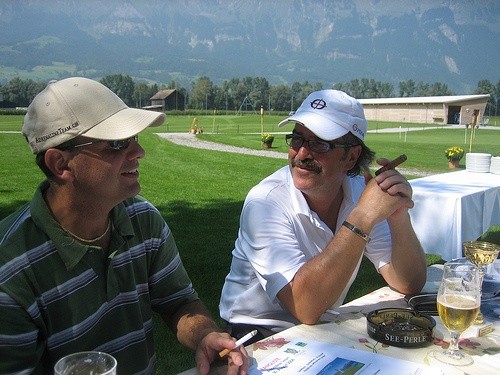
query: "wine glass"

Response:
[465,240,500,296]
[428,258,485,366]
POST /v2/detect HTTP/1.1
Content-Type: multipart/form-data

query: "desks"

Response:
[179,264,500,375]
[408,169,500,261]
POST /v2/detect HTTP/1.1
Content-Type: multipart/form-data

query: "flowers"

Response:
[261,133,274,144]
[445,146,464,159]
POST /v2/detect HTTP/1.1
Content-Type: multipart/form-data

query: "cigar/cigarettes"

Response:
[219,330,258,357]
[374,154,407,175]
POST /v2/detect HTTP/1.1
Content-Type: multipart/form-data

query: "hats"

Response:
[21,76,167,155]
[277,89,368,143]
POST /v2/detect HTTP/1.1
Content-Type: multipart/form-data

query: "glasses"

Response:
[72,134,138,151]
[285,134,353,153]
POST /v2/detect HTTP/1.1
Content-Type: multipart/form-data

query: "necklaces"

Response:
[59,213,111,242]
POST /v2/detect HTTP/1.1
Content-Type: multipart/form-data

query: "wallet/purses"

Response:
[404,291,439,316]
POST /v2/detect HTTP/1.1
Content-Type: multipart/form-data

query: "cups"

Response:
[53,351,119,375]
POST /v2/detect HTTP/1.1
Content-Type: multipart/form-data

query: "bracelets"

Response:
[342,221,371,244]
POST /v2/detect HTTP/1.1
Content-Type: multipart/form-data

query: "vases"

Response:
[263,142,271,148]
[448,160,459,168]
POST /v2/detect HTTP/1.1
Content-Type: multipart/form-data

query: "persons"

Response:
[0,76,249,375]
[219,88,427,347]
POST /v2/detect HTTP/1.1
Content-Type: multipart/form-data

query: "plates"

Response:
[466,153,492,173]
